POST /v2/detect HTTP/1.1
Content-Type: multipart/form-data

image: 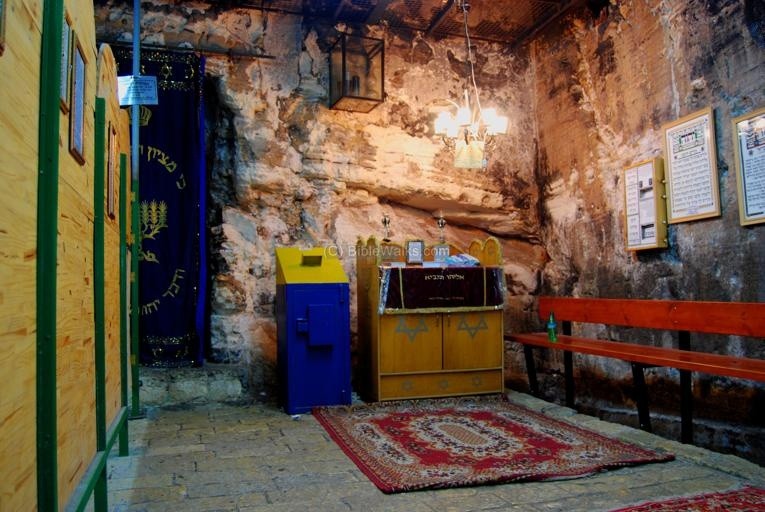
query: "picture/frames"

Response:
[68,27,91,167]
[658,105,724,226]
[58,4,73,115]
[730,106,765,228]
[106,120,117,222]
[405,238,426,267]
[0,0,8,51]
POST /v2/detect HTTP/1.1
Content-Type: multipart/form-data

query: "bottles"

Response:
[546,311,558,344]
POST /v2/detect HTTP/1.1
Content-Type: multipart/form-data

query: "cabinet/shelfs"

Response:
[354,232,508,402]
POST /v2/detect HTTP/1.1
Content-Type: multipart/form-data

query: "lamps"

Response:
[433,0,509,153]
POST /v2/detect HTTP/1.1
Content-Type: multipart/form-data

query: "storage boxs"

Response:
[326,30,387,116]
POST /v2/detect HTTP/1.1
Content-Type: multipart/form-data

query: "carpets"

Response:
[614,478,765,512]
[314,389,676,495]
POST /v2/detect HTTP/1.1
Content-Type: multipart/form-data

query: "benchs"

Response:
[504,292,764,446]
